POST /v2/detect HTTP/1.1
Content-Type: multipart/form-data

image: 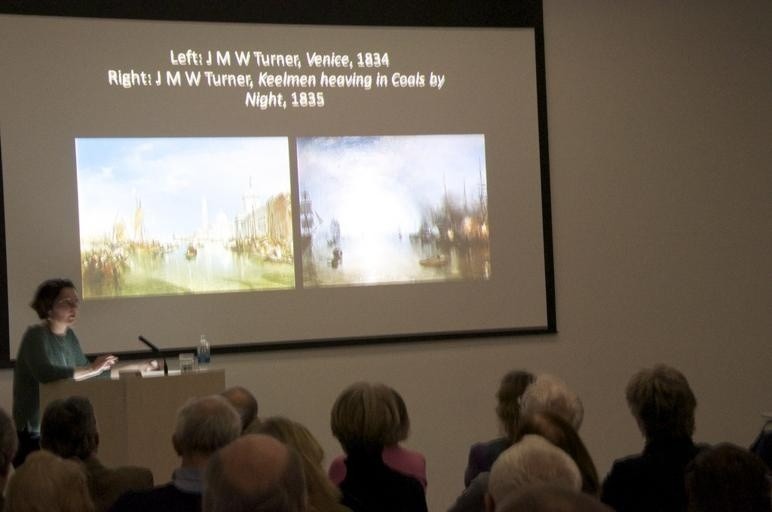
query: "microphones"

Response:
[139,336,168,375]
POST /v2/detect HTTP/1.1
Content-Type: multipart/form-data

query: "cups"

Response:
[178,352,196,376]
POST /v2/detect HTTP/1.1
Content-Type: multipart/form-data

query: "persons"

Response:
[483,435,585,512]
[460,367,537,491]
[598,359,716,510]
[685,440,771,512]
[493,480,618,511]
[444,410,601,510]
[519,371,584,437]
[10,276,120,467]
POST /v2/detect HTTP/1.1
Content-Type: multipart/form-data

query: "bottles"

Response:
[195,332,212,371]
